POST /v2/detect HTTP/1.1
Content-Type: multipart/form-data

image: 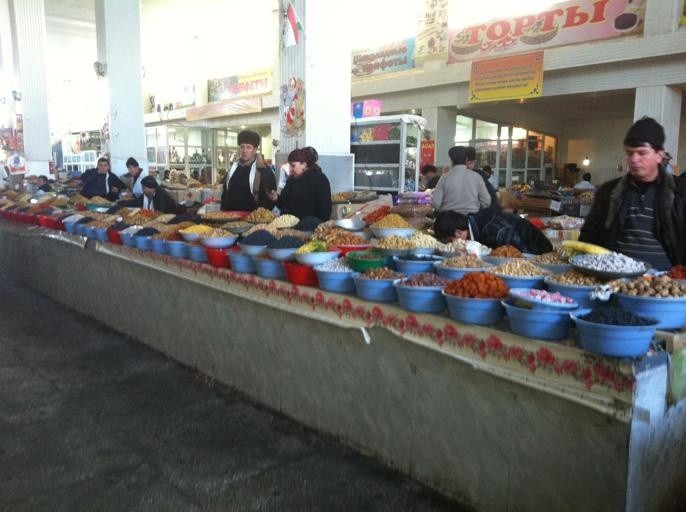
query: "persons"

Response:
[578,116,686,271]
[119,157,147,199]
[272,146,318,216]
[0,165,10,189]
[36,175,53,193]
[481,165,498,193]
[266,149,331,223]
[79,159,125,202]
[664,151,678,175]
[567,164,580,186]
[573,172,594,188]
[465,147,499,214]
[431,147,491,216]
[220,131,277,211]
[422,164,439,190]
[434,209,553,255]
[80,158,107,184]
[117,175,177,214]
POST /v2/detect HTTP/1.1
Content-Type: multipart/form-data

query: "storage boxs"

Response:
[521,193,562,212]
[163,189,201,206]
[561,202,592,218]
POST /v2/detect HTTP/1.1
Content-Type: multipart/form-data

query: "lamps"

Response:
[92,60,105,80]
[11,90,21,102]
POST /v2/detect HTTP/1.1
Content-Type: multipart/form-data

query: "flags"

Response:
[283,2,303,48]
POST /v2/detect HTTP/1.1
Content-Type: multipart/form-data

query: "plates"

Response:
[612,12,639,34]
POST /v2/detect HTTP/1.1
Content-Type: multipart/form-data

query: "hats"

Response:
[466,146,477,162]
[237,130,260,147]
[140,175,158,188]
[622,116,672,163]
[287,146,319,164]
[125,156,139,167]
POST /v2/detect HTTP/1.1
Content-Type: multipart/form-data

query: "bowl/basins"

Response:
[0,191,686,360]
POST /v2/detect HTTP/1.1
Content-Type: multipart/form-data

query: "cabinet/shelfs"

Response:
[348,115,423,196]
